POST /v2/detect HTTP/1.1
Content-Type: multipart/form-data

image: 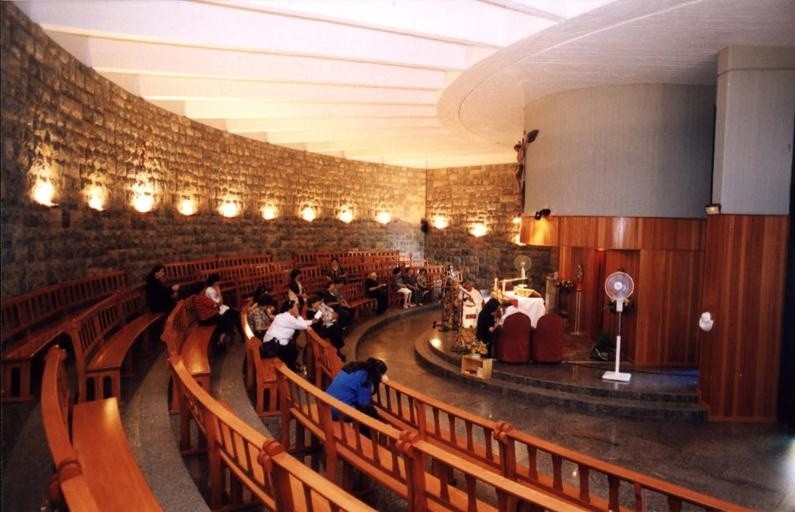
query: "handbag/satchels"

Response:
[260,338,282,359]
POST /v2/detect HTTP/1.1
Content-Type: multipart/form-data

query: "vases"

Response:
[472,353,480,360]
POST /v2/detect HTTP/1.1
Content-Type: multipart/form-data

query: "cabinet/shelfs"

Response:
[461,356,492,380]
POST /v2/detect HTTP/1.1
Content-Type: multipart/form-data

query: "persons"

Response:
[205,273,245,343]
[391,267,415,309]
[192,280,237,346]
[475,297,502,359]
[324,258,350,286]
[312,294,344,337]
[364,271,388,316]
[306,295,347,362]
[416,268,431,301]
[248,296,276,341]
[404,267,424,307]
[253,285,277,312]
[462,282,485,313]
[323,280,358,335]
[263,300,318,373]
[323,357,387,441]
[287,269,309,318]
[499,302,526,328]
[144,264,180,315]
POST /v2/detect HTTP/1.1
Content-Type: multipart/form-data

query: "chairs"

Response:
[534,311,569,364]
[495,312,497,315]
[497,312,532,366]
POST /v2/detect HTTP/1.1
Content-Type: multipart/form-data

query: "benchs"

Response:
[38,344,138,471]
[504,426,756,512]
[158,249,465,321]
[263,442,381,512]
[407,442,594,512]
[239,306,297,415]
[160,295,217,416]
[0,270,132,402]
[306,319,348,388]
[276,364,404,505]
[167,352,275,512]
[69,280,164,407]
[54,461,166,511]
[379,374,503,489]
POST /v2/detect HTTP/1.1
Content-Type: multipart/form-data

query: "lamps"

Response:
[705,204,722,216]
[534,208,550,222]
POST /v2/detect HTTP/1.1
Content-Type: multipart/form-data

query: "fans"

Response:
[601,270,632,382]
[513,252,533,279]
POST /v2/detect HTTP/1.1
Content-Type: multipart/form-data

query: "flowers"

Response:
[471,341,487,355]
